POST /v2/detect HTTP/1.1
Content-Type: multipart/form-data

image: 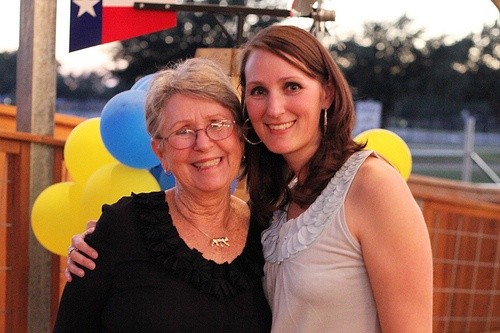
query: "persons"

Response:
[52,59,272,333]
[65,26,434,333]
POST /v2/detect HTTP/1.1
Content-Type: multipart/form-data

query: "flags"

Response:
[68,0,178,52]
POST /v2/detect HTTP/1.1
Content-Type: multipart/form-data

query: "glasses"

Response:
[158,120,236,150]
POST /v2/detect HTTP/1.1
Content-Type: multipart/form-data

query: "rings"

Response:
[68,248,77,256]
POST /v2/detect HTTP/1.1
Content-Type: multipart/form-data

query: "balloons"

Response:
[353,127,412,184]
[30,71,240,256]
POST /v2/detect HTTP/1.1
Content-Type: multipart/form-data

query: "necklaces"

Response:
[178,202,233,248]
